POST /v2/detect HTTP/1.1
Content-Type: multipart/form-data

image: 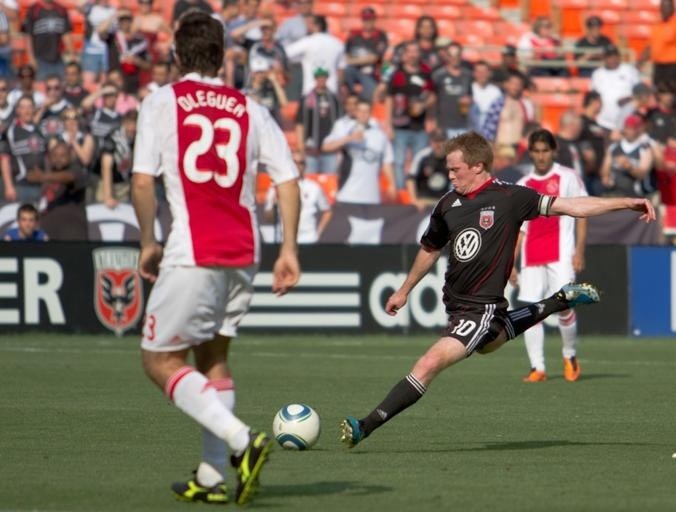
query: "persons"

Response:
[129,12,301,507]
[337,129,659,448]
[1,0,675,245]
[507,130,590,384]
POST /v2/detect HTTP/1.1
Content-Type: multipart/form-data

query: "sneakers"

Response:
[564,357,580,381]
[563,283,599,307]
[170,479,228,503]
[342,418,362,449]
[230,428,272,505]
[524,369,547,381]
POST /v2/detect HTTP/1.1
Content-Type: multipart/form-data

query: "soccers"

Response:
[273,401,322,449]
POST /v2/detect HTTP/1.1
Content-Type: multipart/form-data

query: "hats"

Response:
[313,68,328,76]
[361,7,376,19]
[626,115,644,129]
